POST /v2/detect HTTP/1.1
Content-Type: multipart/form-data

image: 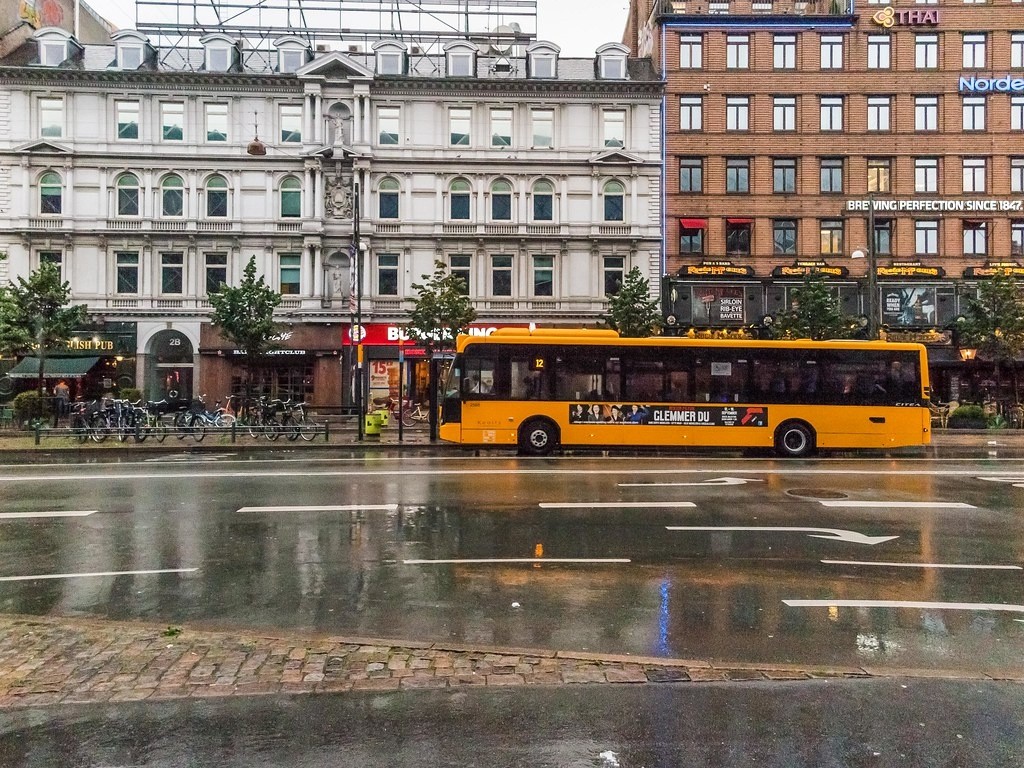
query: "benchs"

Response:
[0,409,14,428]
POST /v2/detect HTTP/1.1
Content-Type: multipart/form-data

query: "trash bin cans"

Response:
[373,406,390,428]
[364,413,382,437]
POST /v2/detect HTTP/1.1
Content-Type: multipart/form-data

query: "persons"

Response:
[572,404,649,425]
[38,379,82,418]
[470,375,488,394]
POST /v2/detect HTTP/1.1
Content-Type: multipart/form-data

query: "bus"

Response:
[434,328,932,457]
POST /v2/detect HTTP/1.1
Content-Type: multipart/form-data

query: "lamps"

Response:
[87,333,97,342]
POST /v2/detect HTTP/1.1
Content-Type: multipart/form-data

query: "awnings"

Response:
[726,218,756,234]
[8,357,99,378]
[976,349,1024,362]
[680,218,708,236]
[928,348,963,366]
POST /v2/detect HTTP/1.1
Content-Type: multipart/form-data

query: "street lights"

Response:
[850,192,876,340]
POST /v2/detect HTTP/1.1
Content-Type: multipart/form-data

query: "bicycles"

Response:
[66,393,319,445]
[387,396,430,427]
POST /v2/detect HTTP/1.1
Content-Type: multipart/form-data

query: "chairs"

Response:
[832,371,903,405]
[694,366,818,404]
[930,402,947,427]
[945,400,959,428]
[985,402,1024,429]
